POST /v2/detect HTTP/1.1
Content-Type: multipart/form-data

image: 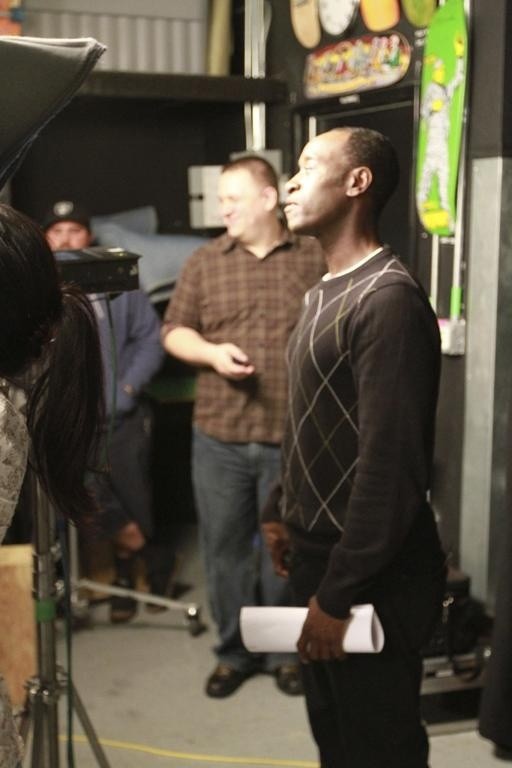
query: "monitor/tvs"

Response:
[290,83,418,279]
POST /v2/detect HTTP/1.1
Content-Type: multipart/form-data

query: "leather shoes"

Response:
[277,662,305,694]
[208,663,248,696]
[112,585,136,620]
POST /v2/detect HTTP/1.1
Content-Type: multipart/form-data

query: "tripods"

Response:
[16,337,110,768]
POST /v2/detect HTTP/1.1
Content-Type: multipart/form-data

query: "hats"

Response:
[43,201,89,231]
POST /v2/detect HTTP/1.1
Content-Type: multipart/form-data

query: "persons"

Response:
[43,195,169,625]
[0,197,112,555]
[160,155,331,696]
[257,121,446,766]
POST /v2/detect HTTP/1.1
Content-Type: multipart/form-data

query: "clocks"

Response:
[315,2,359,38]
